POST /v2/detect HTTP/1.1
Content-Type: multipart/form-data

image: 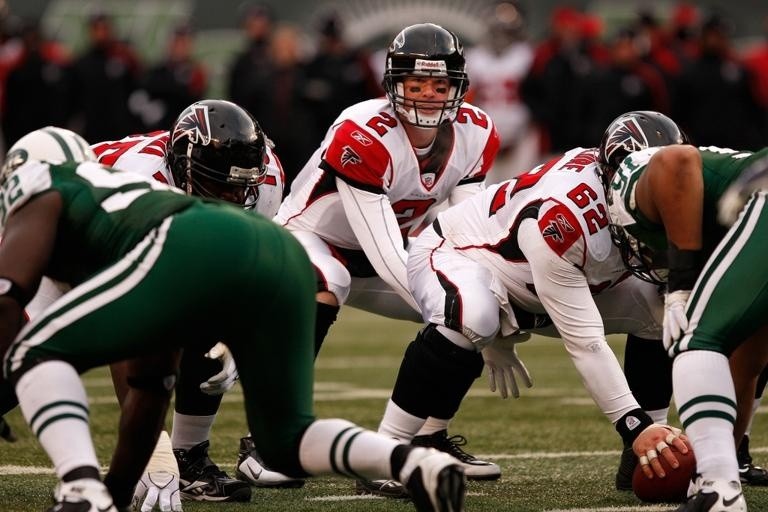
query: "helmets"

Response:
[385,21,469,127]
[163,98,266,213]
[0,124,98,221]
[601,110,686,285]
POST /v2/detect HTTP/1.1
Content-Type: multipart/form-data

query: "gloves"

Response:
[662,290,692,350]
[133,429,183,512]
[480,330,535,400]
[199,341,240,396]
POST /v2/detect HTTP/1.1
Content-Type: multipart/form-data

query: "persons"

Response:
[2,1,768,510]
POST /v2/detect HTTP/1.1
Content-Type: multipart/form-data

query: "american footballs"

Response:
[633,438,696,502]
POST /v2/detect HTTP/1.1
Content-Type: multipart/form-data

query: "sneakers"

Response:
[354,429,501,511]
[613,446,768,512]
[42,478,119,512]
[174,433,310,504]
